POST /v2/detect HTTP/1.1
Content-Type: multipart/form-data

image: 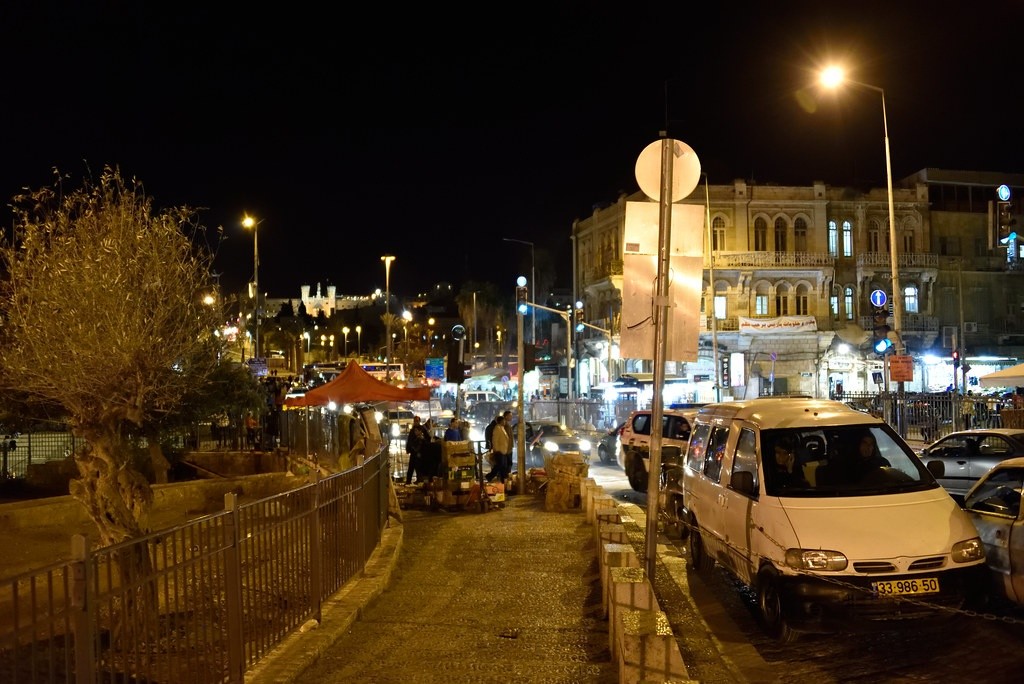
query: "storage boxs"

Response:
[391,441,590,510]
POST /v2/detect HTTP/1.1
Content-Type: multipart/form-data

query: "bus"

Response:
[358,363,405,381]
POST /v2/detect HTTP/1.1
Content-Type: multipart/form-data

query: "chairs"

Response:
[960,438,983,457]
[762,435,846,491]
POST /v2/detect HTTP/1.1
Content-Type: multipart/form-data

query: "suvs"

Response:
[616,410,700,493]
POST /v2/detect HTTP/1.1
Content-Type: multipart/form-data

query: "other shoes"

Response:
[404,482,417,487]
[485,474,492,482]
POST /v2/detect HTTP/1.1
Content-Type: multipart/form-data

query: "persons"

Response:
[842,434,892,473]
[407,409,514,489]
[678,423,688,438]
[768,444,807,492]
[211,374,292,452]
[920,385,1024,442]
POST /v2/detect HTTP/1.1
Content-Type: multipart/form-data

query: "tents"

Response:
[979,362,1024,388]
[283,360,433,483]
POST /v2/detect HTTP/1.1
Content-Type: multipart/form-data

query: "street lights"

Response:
[356,326,361,364]
[243,215,258,358]
[503,237,536,344]
[342,327,348,364]
[817,63,906,439]
[304,332,310,364]
[381,256,396,382]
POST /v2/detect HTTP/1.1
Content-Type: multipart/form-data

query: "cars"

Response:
[873,392,988,423]
[910,428,1024,502]
[286,363,454,440]
[952,456,1024,609]
[512,420,590,467]
[595,420,627,463]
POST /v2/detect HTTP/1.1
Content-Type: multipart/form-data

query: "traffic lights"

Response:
[952,350,960,368]
[873,308,891,353]
[515,286,527,315]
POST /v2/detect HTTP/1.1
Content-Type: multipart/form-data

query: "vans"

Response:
[683,401,994,650]
[461,391,504,414]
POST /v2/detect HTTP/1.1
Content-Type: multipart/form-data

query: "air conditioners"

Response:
[909,314,938,331]
[943,326,957,348]
[964,323,978,332]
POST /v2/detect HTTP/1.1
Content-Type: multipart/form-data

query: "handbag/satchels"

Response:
[485,453,496,466]
[420,443,441,462]
